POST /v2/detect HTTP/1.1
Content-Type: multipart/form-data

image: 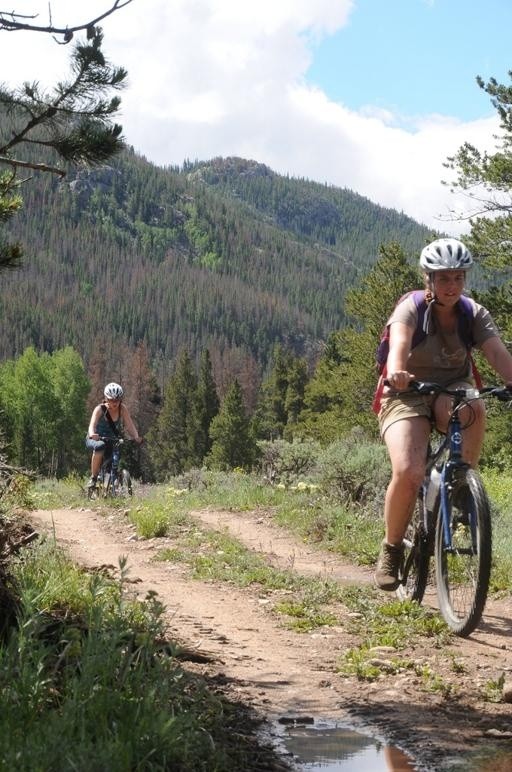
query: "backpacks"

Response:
[371,290,483,414]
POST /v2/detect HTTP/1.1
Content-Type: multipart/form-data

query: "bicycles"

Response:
[377,376,511,636]
[87,435,140,505]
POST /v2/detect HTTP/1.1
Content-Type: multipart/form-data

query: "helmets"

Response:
[420,238,474,274]
[103,383,124,401]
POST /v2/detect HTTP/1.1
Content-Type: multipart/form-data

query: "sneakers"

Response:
[374,538,405,591]
[451,521,472,550]
[88,477,97,488]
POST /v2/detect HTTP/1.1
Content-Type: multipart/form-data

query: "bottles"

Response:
[425,461,446,513]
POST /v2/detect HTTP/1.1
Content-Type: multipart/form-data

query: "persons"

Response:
[87,382,143,488]
[374,237,511,592]
